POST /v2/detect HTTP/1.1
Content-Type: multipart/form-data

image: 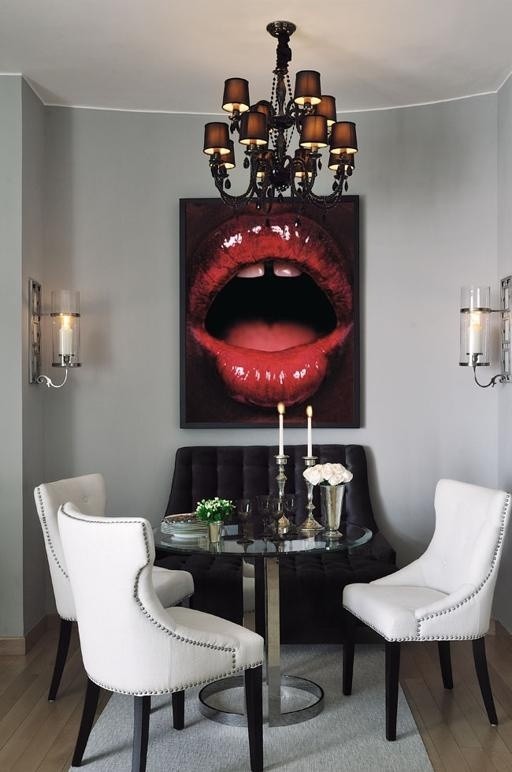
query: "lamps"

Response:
[450,272,511,389]
[24,273,83,390]
[338,476,512,742]
[186,15,360,231]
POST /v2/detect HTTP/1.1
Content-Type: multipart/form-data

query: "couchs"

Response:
[148,441,399,645]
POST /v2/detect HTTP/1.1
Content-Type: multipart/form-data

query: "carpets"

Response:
[70,641,436,772]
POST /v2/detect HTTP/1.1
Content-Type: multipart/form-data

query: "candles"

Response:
[305,404,315,454]
[278,400,286,454]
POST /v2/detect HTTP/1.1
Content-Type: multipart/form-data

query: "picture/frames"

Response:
[177,193,365,428]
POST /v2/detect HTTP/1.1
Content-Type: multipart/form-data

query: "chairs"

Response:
[53,494,268,772]
[31,471,197,702]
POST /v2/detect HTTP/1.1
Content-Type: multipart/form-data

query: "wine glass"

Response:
[256,492,300,544]
[232,499,255,544]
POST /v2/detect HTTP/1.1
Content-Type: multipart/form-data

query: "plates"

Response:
[158,512,213,540]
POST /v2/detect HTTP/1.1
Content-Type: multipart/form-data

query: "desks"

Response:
[149,517,375,729]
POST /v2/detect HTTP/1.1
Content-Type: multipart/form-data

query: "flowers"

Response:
[304,459,355,487]
[195,497,235,520]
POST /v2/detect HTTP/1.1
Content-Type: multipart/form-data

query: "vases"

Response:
[207,521,225,544]
[319,483,351,539]
